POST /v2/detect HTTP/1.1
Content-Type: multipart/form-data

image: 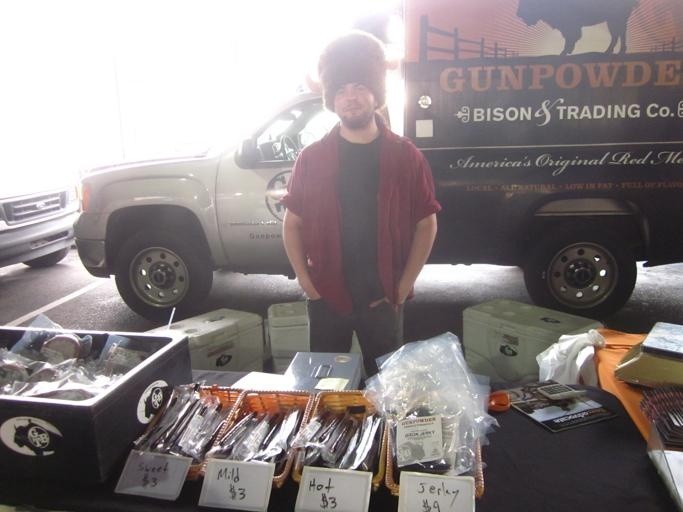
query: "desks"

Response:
[593,330,683,512]
[1,369,678,512]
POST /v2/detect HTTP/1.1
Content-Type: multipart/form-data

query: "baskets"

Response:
[135,381,485,499]
[201,388,315,489]
[136,383,244,481]
[384,420,485,501]
[291,389,388,494]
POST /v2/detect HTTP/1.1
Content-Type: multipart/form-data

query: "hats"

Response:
[319,31,388,111]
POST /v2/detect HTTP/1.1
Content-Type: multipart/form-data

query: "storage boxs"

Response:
[460,297,604,385]
[0,328,194,501]
[140,300,363,391]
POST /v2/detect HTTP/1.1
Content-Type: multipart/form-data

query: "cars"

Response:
[0,159,81,268]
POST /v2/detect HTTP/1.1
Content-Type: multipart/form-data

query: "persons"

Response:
[278,81,443,380]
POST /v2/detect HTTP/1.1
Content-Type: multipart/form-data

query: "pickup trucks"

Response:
[73,53,681,321]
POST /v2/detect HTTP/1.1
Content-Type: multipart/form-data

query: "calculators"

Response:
[538,383,587,400]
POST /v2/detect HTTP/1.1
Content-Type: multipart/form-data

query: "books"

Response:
[502,377,618,437]
[638,381,682,449]
